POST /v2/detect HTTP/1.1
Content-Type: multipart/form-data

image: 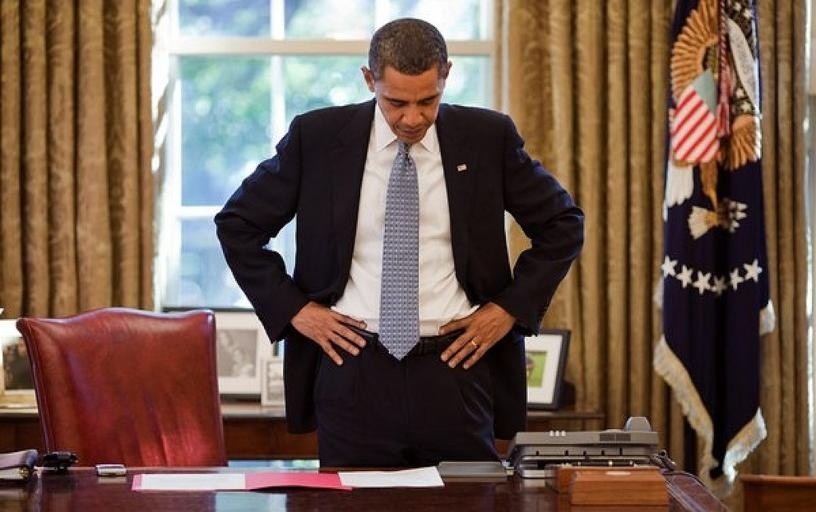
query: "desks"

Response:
[1,465,732,511]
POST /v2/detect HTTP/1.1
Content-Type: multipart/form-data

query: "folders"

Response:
[1,447,39,482]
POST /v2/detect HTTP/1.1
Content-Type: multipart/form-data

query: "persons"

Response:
[211,15,587,467]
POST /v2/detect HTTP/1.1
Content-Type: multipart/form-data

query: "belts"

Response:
[345,325,464,358]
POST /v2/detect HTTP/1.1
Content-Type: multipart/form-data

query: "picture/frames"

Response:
[261,356,285,408]
[163,306,278,404]
[524,327,573,410]
[1,323,37,409]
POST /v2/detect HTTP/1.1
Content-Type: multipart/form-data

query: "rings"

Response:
[471,339,478,349]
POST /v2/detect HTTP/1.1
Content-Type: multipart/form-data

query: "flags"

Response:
[647,0,778,501]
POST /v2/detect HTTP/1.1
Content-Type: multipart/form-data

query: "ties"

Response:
[378,137,420,362]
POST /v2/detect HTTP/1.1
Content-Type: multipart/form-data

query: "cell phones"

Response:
[98,477,127,484]
[95,463,127,477]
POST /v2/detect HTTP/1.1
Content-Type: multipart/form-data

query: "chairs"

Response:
[16,308,228,466]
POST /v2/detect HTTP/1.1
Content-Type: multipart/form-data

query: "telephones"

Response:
[506,417,676,479]
